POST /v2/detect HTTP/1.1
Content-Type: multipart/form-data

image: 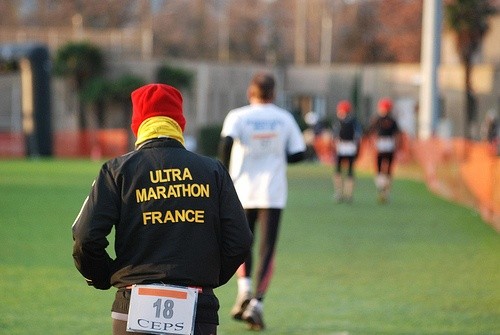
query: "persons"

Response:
[328,98,404,202]
[220,74,307,329]
[72,84,254,335]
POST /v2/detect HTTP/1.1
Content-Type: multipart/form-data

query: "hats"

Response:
[131,84,186,138]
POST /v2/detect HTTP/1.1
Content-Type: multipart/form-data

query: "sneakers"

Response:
[231,290,265,330]
